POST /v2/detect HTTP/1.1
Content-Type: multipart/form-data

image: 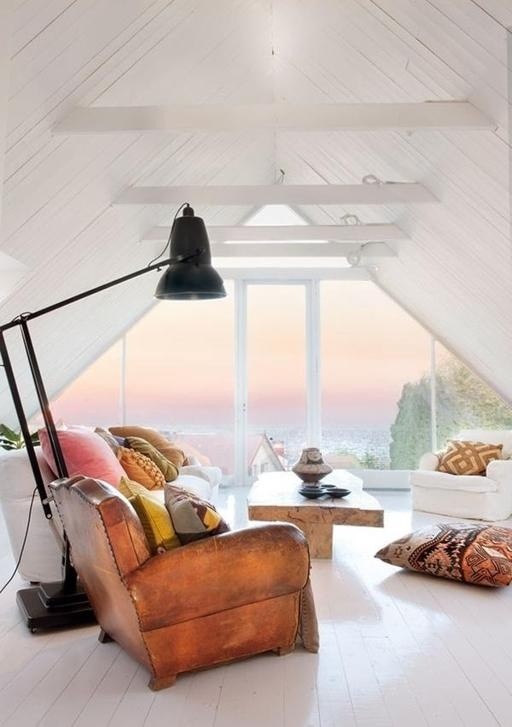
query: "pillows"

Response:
[371,516,512,591]
[34,419,231,554]
[433,435,504,476]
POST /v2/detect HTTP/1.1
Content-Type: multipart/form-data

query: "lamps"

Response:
[0,200,229,636]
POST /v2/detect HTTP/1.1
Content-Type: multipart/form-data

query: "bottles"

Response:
[291,447,333,486]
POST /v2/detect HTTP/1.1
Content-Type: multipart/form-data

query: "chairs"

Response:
[46,474,311,690]
[410,424,511,522]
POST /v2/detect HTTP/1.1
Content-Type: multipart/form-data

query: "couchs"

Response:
[0,437,224,584]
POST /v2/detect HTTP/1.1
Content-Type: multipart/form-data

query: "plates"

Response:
[298,484,352,500]
